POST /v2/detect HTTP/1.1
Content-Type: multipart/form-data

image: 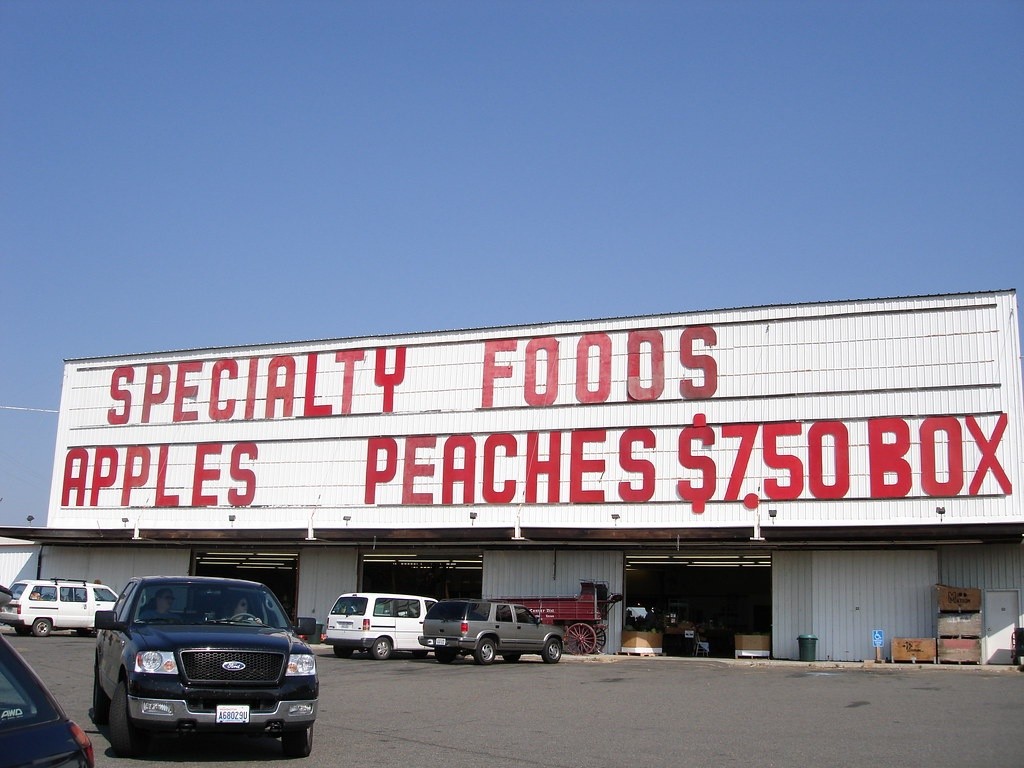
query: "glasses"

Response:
[237,602,249,607]
[161,596,175,600]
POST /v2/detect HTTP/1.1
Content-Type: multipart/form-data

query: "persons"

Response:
[220,596,262,624]
[138,588,183,624]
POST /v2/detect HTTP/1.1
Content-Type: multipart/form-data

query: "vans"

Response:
[0,577,119,637]
[326,593,438,660]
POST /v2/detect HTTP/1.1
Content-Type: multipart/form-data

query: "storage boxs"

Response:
[890,636,936,664]
[936,612,982,638]
[733,635,770,650]
[936,586,982,613]
[936,638,981,664]
[621,630,663,647]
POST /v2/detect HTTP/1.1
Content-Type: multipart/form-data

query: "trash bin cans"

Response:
[307,623,324,644]
[796,634,819,661]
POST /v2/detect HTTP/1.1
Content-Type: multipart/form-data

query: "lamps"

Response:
[748,513,765,540]
[469,512,477,525]
[304,517,318,540]
[935,505,945,521]
[768,509,777,523]
[121,517,129,527]
[510,515,526,539]
[342,515,351,525]
[131,521,143,540]
[610,513,620,525]
[26,514,34,527]
[228,514,236,527]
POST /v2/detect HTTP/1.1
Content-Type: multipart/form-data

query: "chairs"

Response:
[692,633,709,656]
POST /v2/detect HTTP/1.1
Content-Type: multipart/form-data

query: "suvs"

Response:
[418,599,568,665]
[91,575,319,758]
